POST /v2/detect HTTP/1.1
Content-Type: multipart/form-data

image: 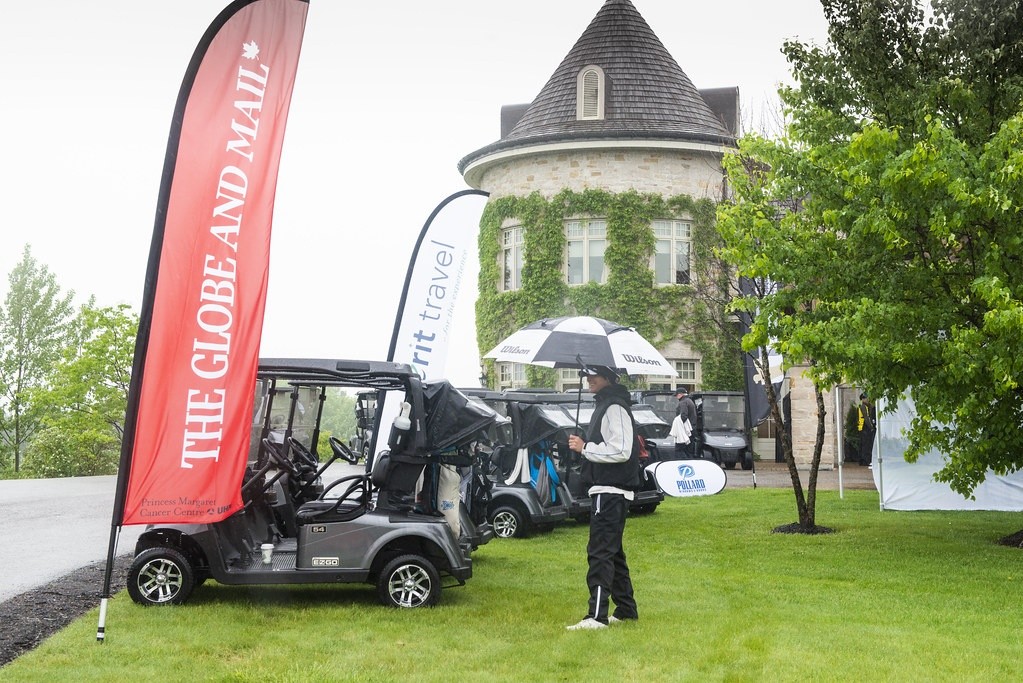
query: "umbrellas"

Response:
[481,308,679,461]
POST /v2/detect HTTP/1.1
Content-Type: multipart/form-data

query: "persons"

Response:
[857,392,876,466]
[565,366,639,630]
[673,387,699,460]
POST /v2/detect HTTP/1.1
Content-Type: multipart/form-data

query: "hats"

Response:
[859,391,869,401]
[672,387,687,397]
[577,365,619,377]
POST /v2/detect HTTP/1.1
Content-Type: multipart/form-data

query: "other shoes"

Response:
[859,462,874,467]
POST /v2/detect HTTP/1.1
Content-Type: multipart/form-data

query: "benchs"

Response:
[290,450,390,525]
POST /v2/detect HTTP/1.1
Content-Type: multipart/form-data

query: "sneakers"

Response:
[564,618,609,633]
[606,614,626,626]
